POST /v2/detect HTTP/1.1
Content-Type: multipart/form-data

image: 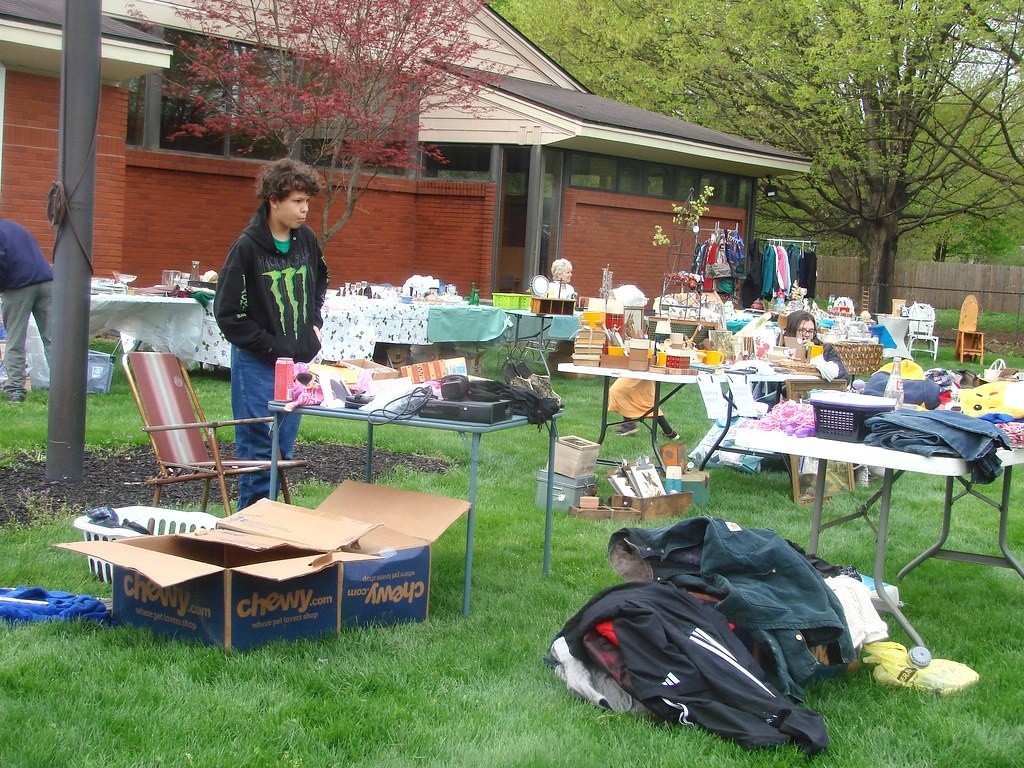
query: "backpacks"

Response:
[546,579,829,753]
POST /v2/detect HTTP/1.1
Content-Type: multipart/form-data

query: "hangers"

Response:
[713,229,818,256]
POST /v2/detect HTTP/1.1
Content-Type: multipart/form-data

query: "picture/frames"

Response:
[786,379,856,504]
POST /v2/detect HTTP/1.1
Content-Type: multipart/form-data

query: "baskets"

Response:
[491,292,533,311]
[810,399,895,443]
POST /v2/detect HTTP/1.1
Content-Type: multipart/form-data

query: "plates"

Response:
[91,284,123,294]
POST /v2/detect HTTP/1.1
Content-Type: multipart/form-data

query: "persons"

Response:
[213,158,330,512]
[547,258,577,300]
[767,309,850,399]
[607,319,680,441]
[0,218,54,402]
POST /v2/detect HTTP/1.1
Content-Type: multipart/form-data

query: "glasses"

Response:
[797,328,816,336]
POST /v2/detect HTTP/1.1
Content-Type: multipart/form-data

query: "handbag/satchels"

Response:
[983,359,1007,382]
[864,641,979,696]
[706,241,747,293]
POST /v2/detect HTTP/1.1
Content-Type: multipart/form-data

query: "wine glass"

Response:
[115,274,137,294]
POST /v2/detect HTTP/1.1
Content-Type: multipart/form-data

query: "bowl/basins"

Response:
[403,297,412,303]
[188,282,216,291]
[607,346,625,356]
[91,277,114,284]
[442,299,458,305]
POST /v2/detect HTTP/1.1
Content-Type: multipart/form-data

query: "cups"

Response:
[162,270,181,288]
[657,352,666,366]
[695,350,724,367]
[578,312,605,329]
[446,284,458,297]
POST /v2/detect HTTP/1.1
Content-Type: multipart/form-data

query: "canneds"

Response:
[274,357,294,402]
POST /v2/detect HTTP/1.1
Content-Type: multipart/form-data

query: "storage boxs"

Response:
[536,433,712,519]
[52,480,472,650]
[810,401,894,441]
[341,355,468,383]
[492,292,531,310]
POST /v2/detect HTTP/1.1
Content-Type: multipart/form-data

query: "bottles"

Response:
[469,281,479,305]
[884,357,904,410]
[828,295,835,303]
[648,347,653,357]
[670,333,683,349]
[665,465,681,494]
[426,291,437,305]
[866,331,878,343]
[336,280,372,298]
[192,260,200,282]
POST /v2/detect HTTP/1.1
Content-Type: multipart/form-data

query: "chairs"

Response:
[908,295,985,363]
[125,350,308,516]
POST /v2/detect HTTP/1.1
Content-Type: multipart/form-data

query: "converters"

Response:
[441,376,469,399]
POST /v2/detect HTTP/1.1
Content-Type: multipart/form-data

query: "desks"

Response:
[879,316,910,359]
[646,317,881,373]
[559,361,820,480]
[735,428,1024,646]
[268,401,563,616]
[90,297,582,374]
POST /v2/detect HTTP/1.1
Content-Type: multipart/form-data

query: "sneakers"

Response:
[662,430,680,440]
[614,423,639,435]
